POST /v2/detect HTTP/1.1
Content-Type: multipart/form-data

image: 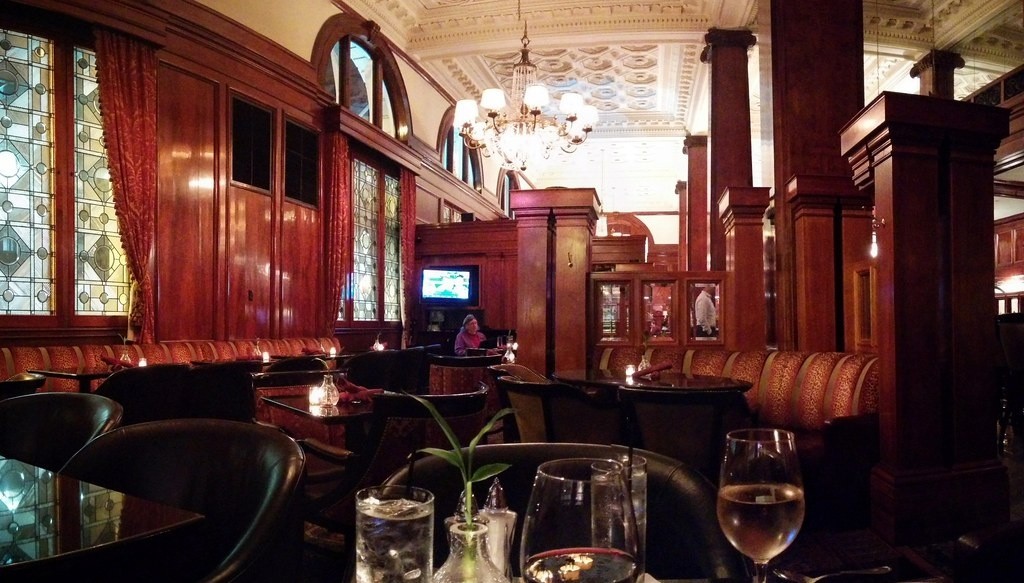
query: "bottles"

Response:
[443,486,490,548]
[478,477,518,583]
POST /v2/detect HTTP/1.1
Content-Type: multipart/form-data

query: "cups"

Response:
[519,457,640,583]
[590,453,648,583]
[354,485,435,583]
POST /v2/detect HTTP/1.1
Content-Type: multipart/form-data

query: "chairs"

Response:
[0,343,741,583]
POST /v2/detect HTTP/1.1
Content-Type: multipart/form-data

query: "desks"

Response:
[0,459,205,572]
[26,364,112,393]
[264,393,373,455]
[551,368,753,441]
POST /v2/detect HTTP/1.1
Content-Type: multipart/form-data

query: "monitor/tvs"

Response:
[419,268,474,303]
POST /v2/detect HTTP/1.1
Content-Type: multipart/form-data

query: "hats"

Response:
[462,314,474,326]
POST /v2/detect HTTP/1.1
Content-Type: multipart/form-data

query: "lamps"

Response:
[452,21,601,170]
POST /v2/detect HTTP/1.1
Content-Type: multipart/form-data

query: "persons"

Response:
[455,315,487,357]
[694,286,717,337]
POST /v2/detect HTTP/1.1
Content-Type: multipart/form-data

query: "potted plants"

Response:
[397,390,519,583]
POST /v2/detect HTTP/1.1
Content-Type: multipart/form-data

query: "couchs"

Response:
[592,345,881,453]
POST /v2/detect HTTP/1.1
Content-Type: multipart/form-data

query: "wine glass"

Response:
[718,428,806,583]
